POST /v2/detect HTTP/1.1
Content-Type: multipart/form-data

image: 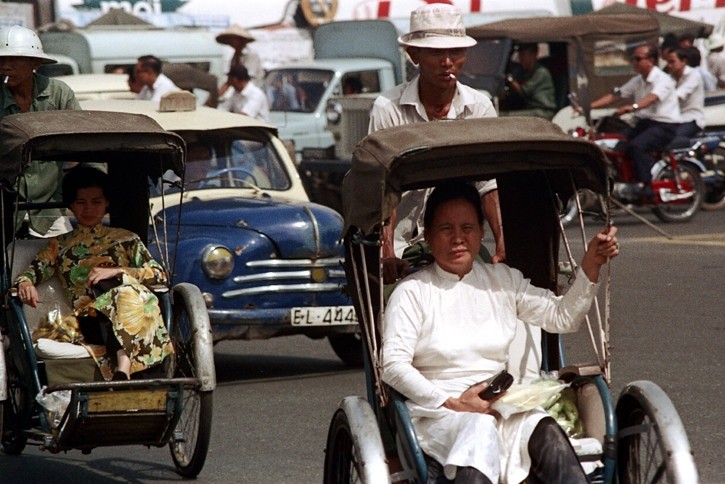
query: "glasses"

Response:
[631,56,649,62]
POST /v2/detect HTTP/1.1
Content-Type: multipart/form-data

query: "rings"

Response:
[615,243,619,249]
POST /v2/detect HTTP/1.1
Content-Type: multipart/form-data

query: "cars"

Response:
[31,11,725,370]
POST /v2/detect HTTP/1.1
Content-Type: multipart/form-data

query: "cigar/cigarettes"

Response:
[450,73,456,79]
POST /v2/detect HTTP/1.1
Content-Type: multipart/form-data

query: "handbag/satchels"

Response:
[462,370,514,400]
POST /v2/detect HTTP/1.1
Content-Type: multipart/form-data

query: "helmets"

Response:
[0,25,57,64]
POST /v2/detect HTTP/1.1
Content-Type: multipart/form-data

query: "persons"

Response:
[226,64,269,150]
[378,182,619,484]
[660,34,725,138]
[343,77,363,94]
[504,44,555,118]
[216,22,265,95]
[368,3,505,286]
[270,73,300,110]
[287,74,306,109]
[135,55,176,99]
[590,44,680,208]
[0,25,106,238]
[11,164,176,391]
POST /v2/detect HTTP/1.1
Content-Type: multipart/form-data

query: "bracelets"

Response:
[508,76,513,81]
[632,103,638,111]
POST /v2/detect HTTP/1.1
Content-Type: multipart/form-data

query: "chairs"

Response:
[10,242,116,385]
[381,279,605,483]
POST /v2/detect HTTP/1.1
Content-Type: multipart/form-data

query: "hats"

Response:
[227,63,250,79]
[216,24,255,43]
[398,3,478,49]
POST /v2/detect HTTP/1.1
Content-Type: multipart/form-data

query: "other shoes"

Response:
[621,185,649,195]
[113,370,132,391]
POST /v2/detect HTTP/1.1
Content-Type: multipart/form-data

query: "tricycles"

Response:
[320,114,703,484]
[0,109,219,483]
